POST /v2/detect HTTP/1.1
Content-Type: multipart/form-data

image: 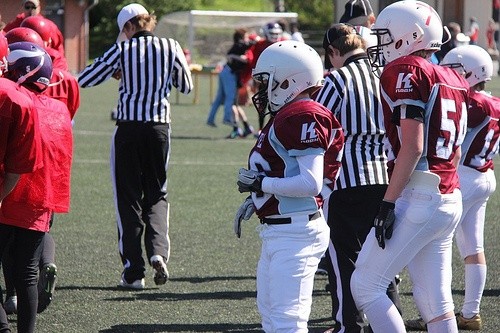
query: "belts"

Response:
[260,211,321,224]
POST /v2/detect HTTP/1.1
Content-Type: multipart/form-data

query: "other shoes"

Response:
[242,125,253,137]
[206,121,218,128]
[229,127,243,138]
[455,313,482,330]
[404,317,428,331]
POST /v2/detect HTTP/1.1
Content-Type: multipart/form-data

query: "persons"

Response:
[435,22,460,60]
[439,44,500,328]
[0,0,79,333]
[466,17,478,45]
[354,25,400,290]
[350,0,468,333]
[208,22,303,138]
[315,24,401,333]
[487,19,499,56]
[339,0,375,30]
[78,3,194,289]
[235,40,344,333]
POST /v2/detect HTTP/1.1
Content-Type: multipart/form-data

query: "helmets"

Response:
[366,0,444,79]
[251,40,326,119]
[0,33,9,79]
[20,16,52,42]
[353,26,378,58]
[265,23,283,43]
[4,41,52,94]
[4,27,44,51]
[438,45,493,88]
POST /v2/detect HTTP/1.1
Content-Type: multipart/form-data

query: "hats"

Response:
[323,23,360,69]
[339,0,373,23]
[116,4,149,43]
[24,0,40,8]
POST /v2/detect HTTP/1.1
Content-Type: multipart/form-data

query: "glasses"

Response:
[25,6,37,10]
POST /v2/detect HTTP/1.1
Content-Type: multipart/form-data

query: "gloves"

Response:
[237,168,267,194]
[374,200,396,249]
[233,195,255,238]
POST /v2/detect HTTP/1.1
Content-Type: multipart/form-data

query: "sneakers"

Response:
[3,295,17,314]
[36,263,58,314]
[150,255,169,286]
[120,275,145,289]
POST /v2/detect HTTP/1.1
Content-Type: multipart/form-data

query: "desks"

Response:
[176,70,220,105]
